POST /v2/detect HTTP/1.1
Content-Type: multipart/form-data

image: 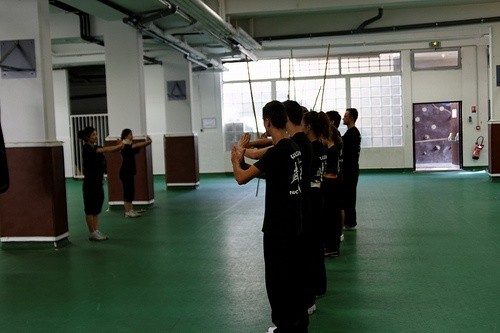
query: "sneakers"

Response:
[308,304,316,314]
[342,225,357,230]
[324,248,340,256]
[125,210,142,217]
[267,326,277,333]
[89,230,108,240]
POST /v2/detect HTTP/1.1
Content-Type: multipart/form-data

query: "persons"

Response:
[119,129,152,218]
[245,100,345,259]
[231,100,310,333]
[340,108,361,230]
[237,100,317,315]
[78,126,125,240]
